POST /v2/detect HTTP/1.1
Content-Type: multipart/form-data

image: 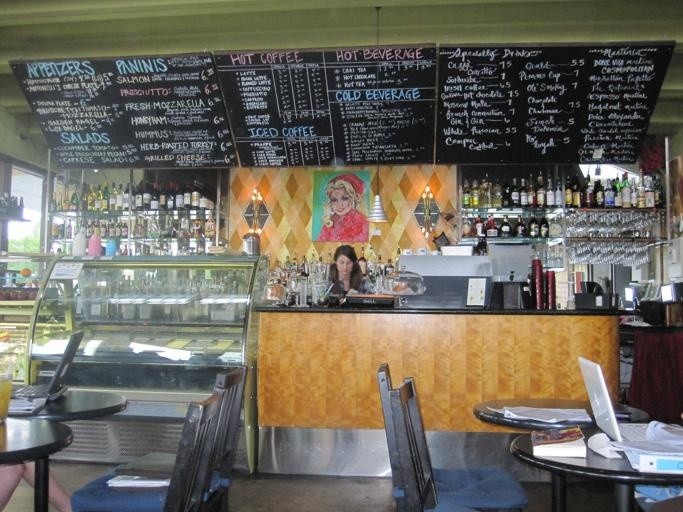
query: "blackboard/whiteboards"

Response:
[8,57,143,170]
[109,58,239,169]
[322,49,436,165]
[210,52,336,167]
[440,41,675,163]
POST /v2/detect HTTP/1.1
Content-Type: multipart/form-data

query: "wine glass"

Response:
[553,211,664,238]
[563,236,651,269]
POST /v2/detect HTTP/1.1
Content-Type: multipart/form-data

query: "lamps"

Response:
[365,7,389,223]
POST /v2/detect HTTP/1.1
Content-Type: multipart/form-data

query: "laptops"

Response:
[10,330,85,398]
[578,356,683,442]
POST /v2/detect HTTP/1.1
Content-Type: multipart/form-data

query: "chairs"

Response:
[71,364,249,510]
[378,364,528,510]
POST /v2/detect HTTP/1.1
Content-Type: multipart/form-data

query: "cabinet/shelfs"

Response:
[457,163,667,239]
[0,164,268,477]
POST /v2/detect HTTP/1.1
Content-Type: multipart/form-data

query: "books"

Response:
[530,427,588,458]
[105,475,176,487]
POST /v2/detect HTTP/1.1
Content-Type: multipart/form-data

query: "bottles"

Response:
[48,176,244,295]
[463,213,551,239]
[462,167,664,209]
[268,246,401,309]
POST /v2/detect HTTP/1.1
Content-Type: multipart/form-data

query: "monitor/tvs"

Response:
[346,294,394,307]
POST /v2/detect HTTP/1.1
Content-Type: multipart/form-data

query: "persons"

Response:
[321,244,372,305]
[315,173,368,242]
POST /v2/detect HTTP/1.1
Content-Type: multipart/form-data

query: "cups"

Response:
[1,368,15,425]
[402,247,441,256]
[530,259,556,311]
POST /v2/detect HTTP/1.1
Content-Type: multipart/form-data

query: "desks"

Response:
[473,392,649,512]
[0,417,73,512]
[510,423,683,511]
